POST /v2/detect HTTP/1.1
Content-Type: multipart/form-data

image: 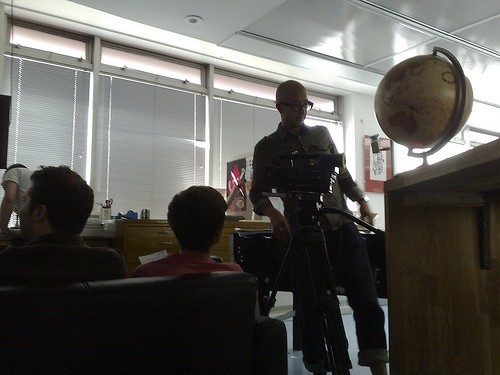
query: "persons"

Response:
[0,166,127,289]
[121,185,245,277]
[0,164,41,243]
[250,80,391,375]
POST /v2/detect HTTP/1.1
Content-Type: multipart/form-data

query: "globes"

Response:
[374,47,473,168]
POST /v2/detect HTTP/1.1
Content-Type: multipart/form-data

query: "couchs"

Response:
[0,271,289,375]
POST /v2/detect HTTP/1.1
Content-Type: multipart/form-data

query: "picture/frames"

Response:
[363,135,394,194]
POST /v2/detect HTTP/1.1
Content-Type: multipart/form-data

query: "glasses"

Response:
[279,101,314,112]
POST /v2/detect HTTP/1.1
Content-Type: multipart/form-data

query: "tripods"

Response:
[261,209,350,375]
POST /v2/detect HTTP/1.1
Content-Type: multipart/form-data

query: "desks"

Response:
[227,230,388,320]
[382,138,500,375]
[114,219,272,277]
[0,236,115,247]
